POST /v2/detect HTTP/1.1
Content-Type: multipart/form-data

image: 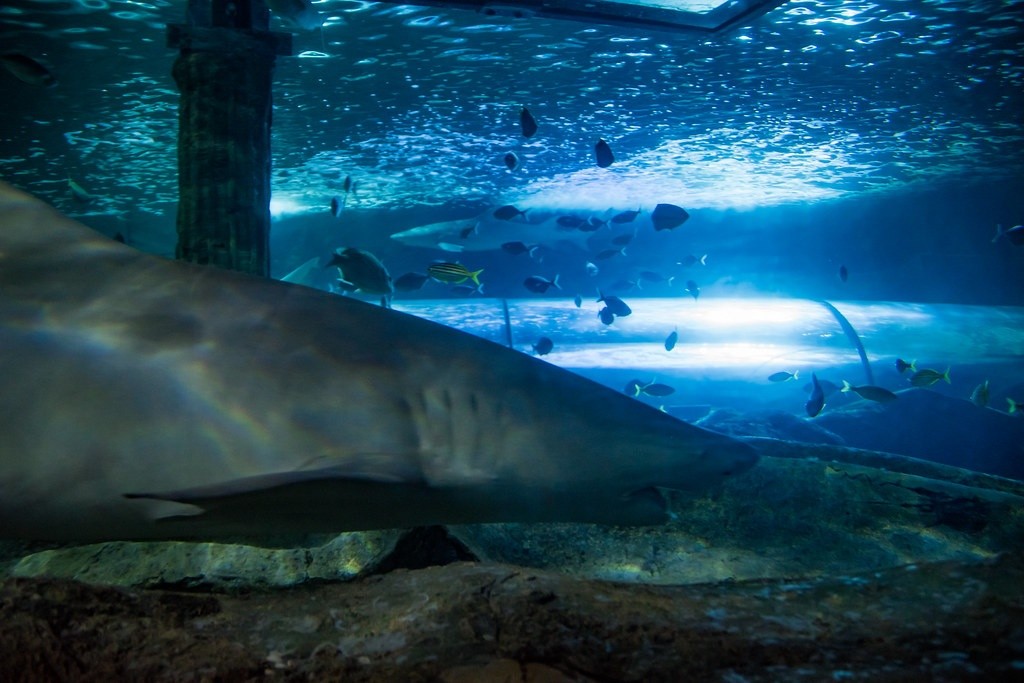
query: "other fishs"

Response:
[0,0,1023,682]
